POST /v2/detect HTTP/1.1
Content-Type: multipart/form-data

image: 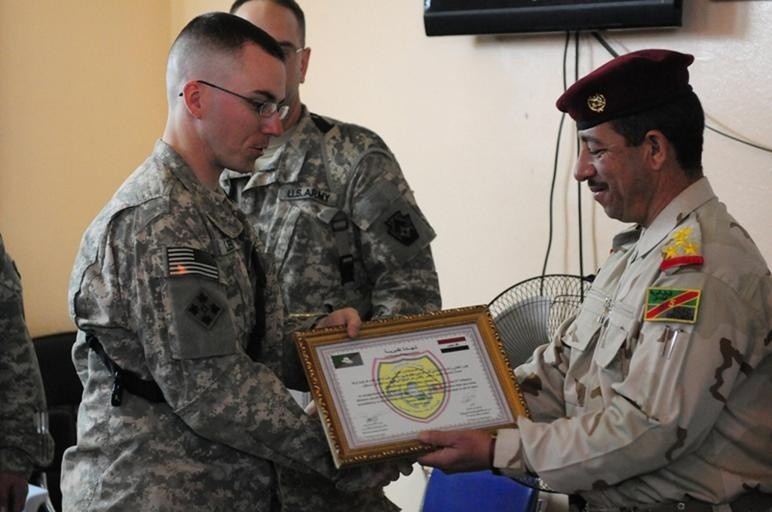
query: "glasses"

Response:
[178,79,290,121]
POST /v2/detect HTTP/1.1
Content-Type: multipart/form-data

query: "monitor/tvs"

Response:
[423,0,684,37]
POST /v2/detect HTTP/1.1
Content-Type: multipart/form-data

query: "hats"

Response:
[555,49,694,130]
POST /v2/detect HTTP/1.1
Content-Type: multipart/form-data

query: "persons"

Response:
[0,231,55,512]
[217,0,442,419]
[59,12,448,512]
[413,49,772,511]
